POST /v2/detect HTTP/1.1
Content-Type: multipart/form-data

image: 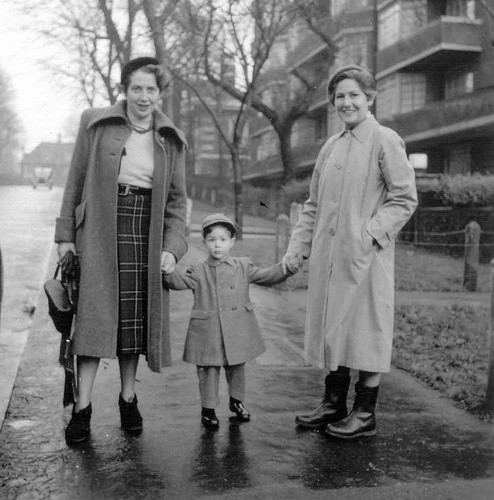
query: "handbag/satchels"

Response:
[43,260,77,335]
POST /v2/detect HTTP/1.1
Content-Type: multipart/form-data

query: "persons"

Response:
[55,56,188,444]
[163,212,298,431]
[282,65,419,439]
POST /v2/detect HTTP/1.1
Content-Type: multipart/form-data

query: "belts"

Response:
[117,183,152,197]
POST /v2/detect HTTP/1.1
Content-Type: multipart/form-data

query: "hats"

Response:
[203,212,237,235]
[121,57,160,84]
[325,65,367,105]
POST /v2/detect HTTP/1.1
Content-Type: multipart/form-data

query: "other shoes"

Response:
[118,391,143,431]
[230,399,250,420]
[65,402,93,443]
[201,407,219,425]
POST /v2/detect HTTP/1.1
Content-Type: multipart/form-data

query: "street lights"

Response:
[408,152,428,248]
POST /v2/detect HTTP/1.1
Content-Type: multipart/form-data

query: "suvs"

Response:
[32,166,54,189]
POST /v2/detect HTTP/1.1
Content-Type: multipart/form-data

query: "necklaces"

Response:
[125,113,154,134]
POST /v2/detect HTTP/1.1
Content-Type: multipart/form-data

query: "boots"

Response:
[326,381,380,439]
[295,373,351,428]
